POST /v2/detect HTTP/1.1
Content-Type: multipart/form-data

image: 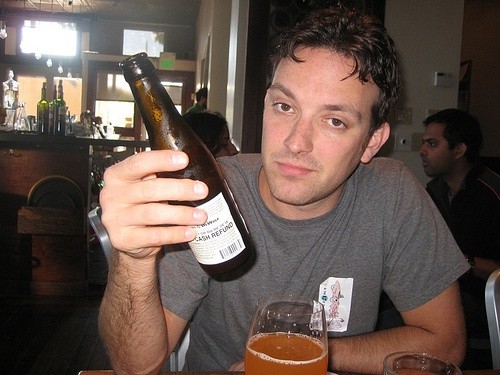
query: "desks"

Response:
[0,132,151,299]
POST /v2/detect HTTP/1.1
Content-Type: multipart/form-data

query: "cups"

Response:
[383,351,463,375]
[244,294,327,375]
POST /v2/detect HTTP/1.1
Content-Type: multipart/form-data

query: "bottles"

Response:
[37,80,66,136]
[118,52,258,283]
[14,102,30,132]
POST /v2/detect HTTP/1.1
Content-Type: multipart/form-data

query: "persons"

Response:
[186,87,207,113]
[419,108,500,276]
[182,111,238,159]
[99,7,470,375]
